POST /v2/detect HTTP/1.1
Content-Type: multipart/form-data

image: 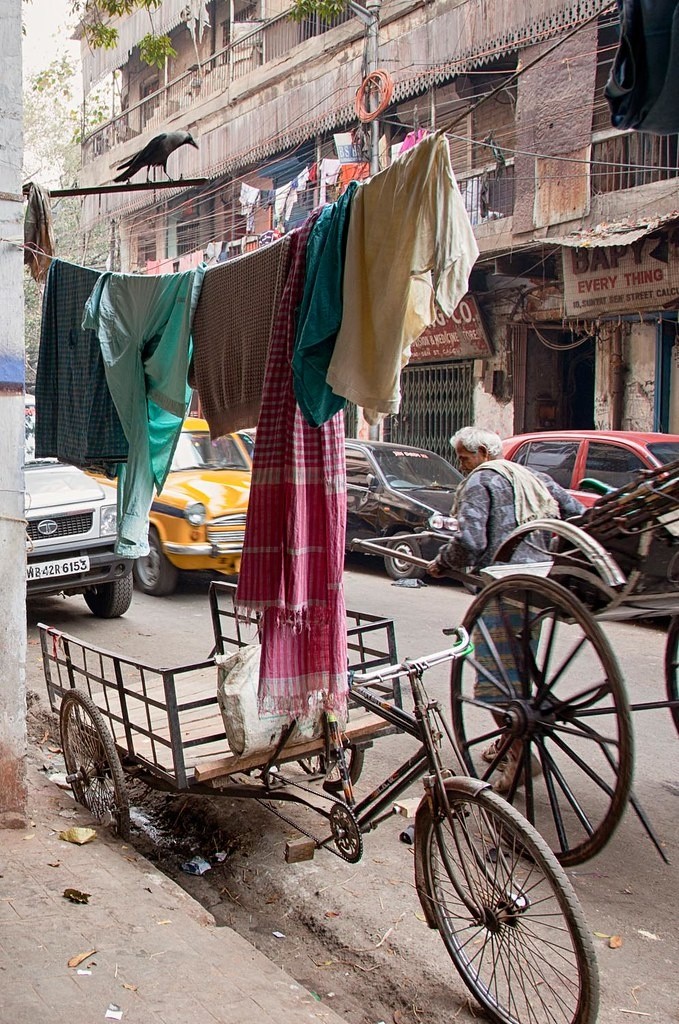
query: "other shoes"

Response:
[493,749,541,792]
[480,744,507,771]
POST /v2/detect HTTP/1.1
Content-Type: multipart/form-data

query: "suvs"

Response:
[23,394,134,619]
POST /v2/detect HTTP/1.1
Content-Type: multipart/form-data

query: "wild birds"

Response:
[112,131,200,183]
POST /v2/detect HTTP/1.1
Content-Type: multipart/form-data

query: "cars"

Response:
[82,416,252,598]
[497,431,679,539]
[344,436,467,582]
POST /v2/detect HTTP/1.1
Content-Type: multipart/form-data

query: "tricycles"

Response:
[36,579,603,1024]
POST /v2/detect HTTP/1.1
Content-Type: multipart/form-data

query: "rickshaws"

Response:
[349,460,679,869]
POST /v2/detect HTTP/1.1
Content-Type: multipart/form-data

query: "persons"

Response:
[426,427,589,791]
